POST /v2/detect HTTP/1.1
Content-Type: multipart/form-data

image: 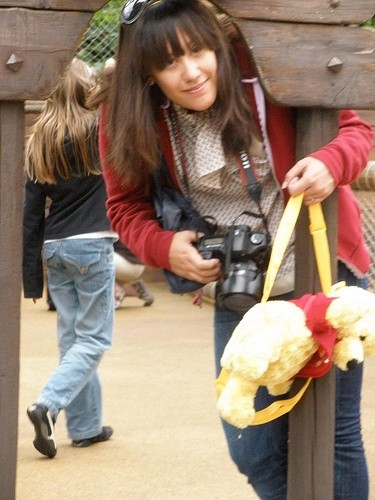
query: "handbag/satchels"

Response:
[213,186,331,431]
[103,123,219,295]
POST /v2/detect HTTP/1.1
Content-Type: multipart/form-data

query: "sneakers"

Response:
[27,405,58,459]
[72,426,113,447]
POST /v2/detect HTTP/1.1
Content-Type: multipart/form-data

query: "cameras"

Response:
[191,224,273,316]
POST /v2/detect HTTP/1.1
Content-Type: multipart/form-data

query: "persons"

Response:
[112,239,156,314]
[18,58,125,459]
[99,0,374,500]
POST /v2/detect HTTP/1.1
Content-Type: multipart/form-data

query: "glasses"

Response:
[120,0,160,25]
